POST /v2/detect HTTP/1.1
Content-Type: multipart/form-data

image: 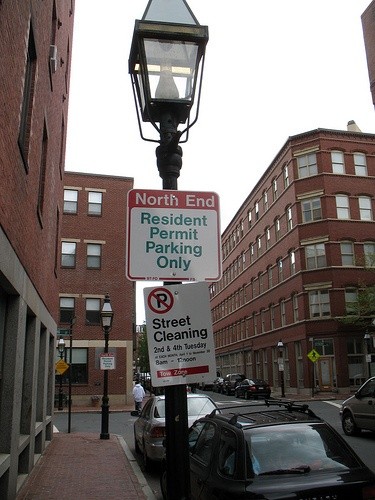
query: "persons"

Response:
[132,381,146,410]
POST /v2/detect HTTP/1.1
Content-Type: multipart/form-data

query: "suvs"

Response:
[160,400,374,500]
[223,373,244,395]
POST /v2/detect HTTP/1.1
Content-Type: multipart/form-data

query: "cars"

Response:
[130,394,222,466]
[234,378,270,399]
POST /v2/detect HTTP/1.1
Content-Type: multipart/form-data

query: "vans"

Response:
[339,376,374,435]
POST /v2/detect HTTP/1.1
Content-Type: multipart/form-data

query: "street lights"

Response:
[58,335,65,410]
[128,0,208,500]
[100,292,113,439]
[277,339,285,398]
